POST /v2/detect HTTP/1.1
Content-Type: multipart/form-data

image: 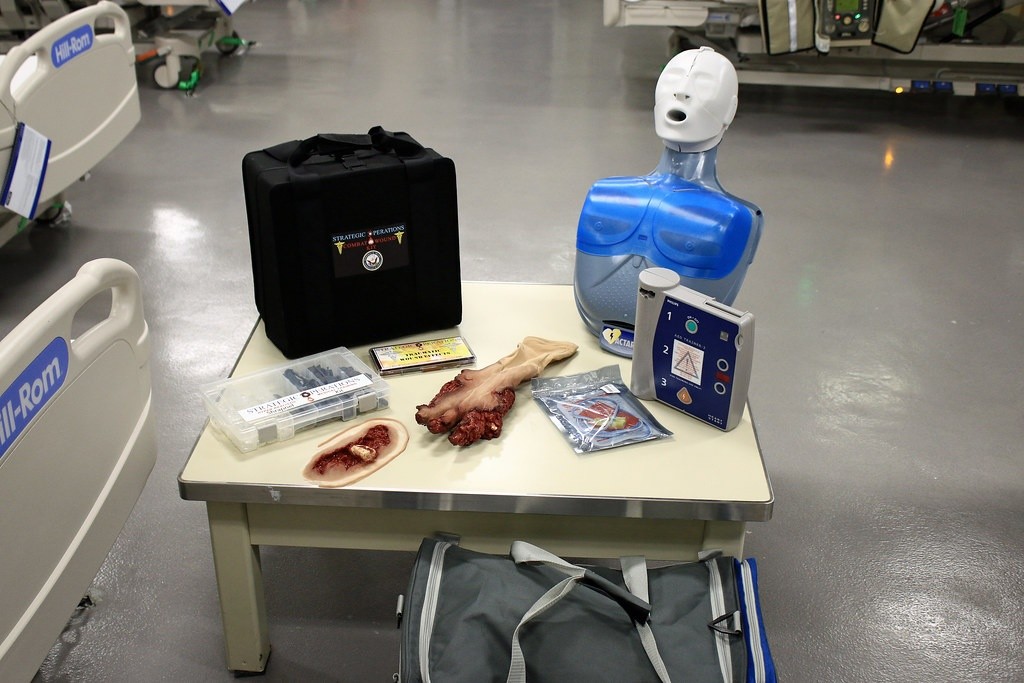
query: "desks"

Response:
[169,276,780,673]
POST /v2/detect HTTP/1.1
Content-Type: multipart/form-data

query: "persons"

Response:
[573,47,764,358]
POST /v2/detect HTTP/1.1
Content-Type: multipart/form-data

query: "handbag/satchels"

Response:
[394,537,780,683]
[240,126,462,359]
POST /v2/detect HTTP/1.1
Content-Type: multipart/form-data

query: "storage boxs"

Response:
[193,345,395,455]
